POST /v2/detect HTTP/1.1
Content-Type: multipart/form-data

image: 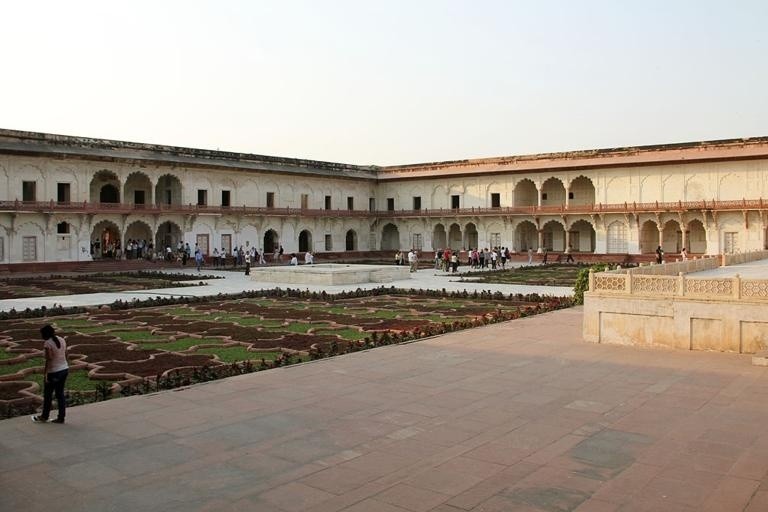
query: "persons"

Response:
[655,245,663,264]
[562,244,574,262]
[676,247,687,261]
[89,235,314,277]
[29,323,69,424]
[394,244,548,273]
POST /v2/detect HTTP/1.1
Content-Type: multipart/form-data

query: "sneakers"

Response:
[31,416,49,422]
[49,419,64,424]
